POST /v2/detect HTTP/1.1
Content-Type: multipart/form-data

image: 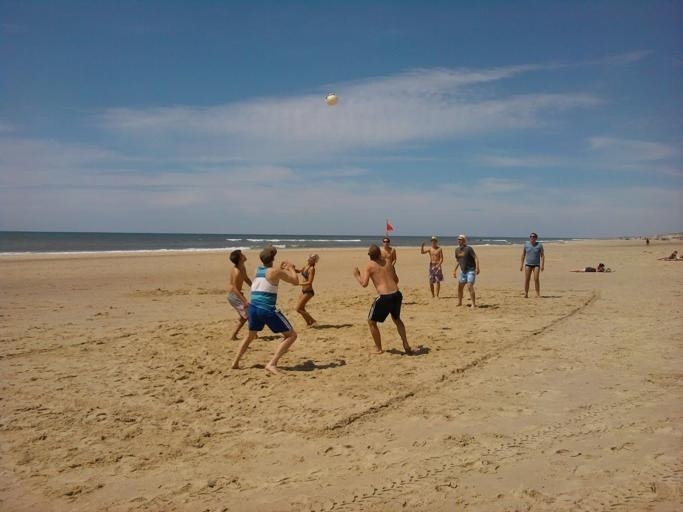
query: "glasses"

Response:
[530,236,536,238]
[383,241,389,243]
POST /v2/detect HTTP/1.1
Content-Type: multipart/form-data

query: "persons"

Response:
[352,244,411,356]
[656,250,678,260]
[568,263,604,273]
[229,245,300,375]
[519,233,545,299]
[378,237,400,290]
[420,236,443,298]
[226,250,257,340]
[292,254,319,327]
[451,235,479,308]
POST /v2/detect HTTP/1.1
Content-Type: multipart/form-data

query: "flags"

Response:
[385,223,393,232]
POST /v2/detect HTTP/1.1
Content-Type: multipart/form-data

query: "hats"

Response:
[431,236,437,241]
[457,235,465,242]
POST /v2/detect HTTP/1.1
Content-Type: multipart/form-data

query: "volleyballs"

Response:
[326,95,338,105]
